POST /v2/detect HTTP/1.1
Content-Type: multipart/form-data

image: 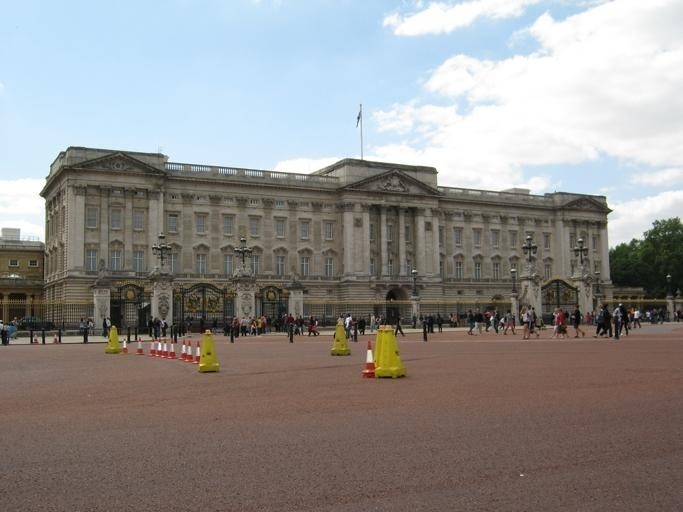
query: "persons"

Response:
[103,317,112,338]
[147,316,167,338]
[412,312,458,342]
[185,316,218,335]
[223,313,320,344]
[375,314,406,337]
[79,318,95,337]
[338,313,366,342]
[466,304,682,341]
[0,317,18,345]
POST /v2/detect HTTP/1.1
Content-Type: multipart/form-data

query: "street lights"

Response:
[594,271,602,293]
[409,266,419,296]
[573,238,588,266]
[233,234,253,266]
[521,235,537,263]
[509,266,519,293]
[152,230,172,267]
[666,273,673,296]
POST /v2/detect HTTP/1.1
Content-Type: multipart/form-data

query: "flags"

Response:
[356,112,361,126]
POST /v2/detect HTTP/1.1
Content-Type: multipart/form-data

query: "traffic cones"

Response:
[52,333,58,344]
[134,335,201,365]
[32,333,40,345]
[121,338,128,354]
[362,339,378,378]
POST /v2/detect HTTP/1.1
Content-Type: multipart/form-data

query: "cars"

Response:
[14,315,53,331]
